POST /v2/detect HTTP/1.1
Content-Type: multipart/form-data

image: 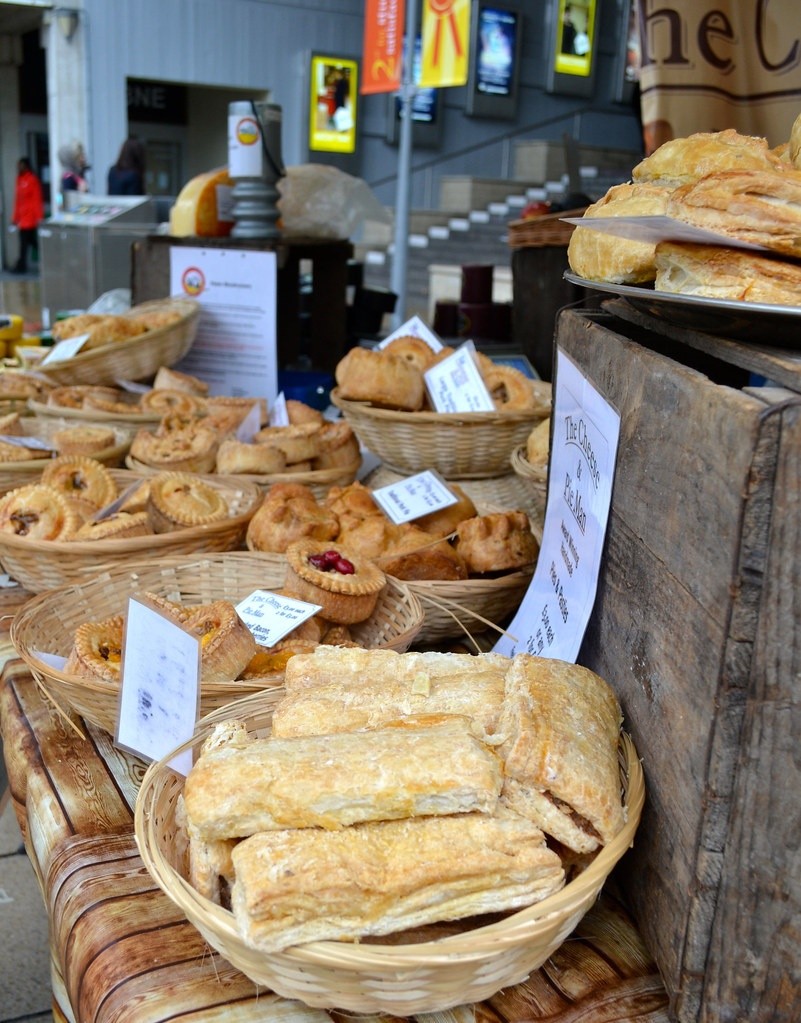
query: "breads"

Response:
[0,312,629,951]
[568,112,801,304]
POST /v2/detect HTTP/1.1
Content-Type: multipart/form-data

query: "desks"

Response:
[426,265,510,329]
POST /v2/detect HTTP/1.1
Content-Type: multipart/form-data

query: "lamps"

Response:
[56,7,80,44]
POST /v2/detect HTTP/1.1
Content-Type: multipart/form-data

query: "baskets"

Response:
[1,299,647,639]
[14,550,425,736]
[133,683,652,1016]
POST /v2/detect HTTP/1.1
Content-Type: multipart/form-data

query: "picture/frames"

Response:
[547,0,602,99]
[301,47,362,163]
[617,0,641,106]
[467,0,522,123]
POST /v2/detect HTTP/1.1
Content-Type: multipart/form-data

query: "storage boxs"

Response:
[131,234,400,402]
[546,298,801,1022]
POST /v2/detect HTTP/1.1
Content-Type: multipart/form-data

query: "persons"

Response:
[562,6,577,54]
[323,66,350,110]
[58,141,89,192]
[108,136,146,195]
[8,157,43,274]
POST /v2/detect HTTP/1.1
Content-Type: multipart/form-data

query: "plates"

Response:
[564,268,800,342]
